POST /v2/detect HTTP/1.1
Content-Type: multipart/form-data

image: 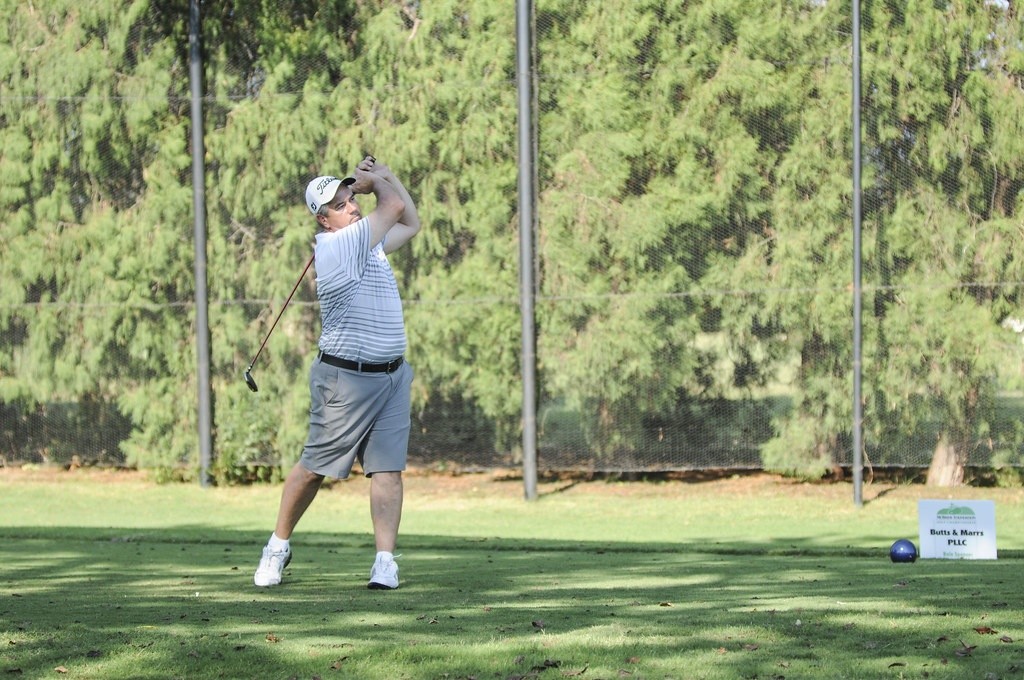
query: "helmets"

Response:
[890,539,917,563]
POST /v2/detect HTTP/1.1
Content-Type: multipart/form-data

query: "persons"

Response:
[254,156,421,588]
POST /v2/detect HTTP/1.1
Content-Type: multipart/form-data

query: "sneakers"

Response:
[366,553,403,588]
[254,542,292,586]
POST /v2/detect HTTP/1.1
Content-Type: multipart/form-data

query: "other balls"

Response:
[889,539,917,563]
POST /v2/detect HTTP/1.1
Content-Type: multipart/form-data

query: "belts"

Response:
[317,349,403,374]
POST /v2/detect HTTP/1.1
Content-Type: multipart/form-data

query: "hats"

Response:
[305,176,356,215]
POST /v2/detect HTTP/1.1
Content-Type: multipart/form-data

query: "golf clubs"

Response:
[244,155,377,393]
